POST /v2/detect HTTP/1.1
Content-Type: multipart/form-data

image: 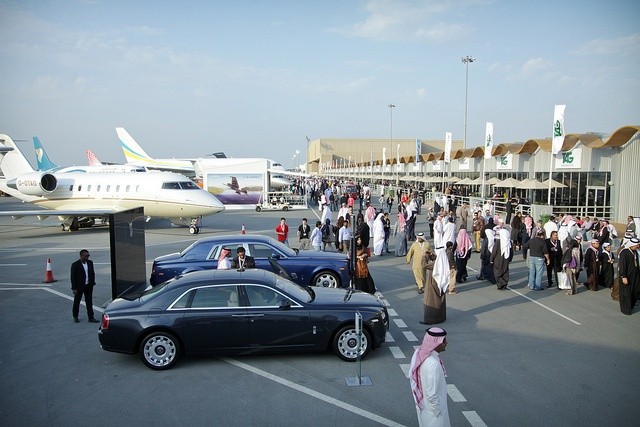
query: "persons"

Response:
[335,216,345,249]
[372,212,386,255]
[393,211,410,256]
[483,199,494,215]
[440,210,449,232]
[588,217,600,237]
[347,236,377,295]
[493,190,501,200]
[438,206,445,216]
[515,195,523,204]
[472,201,483,217]
[573,216,583,228]
[484,209,493,226]
[531,219,546,241]
[337,202,350,221]
[476,230,496,284]
[603,221,618,241]
[452,228,473,283]
[579,216,593,241]
[396,184,427,215]
[433,213,444,249]
[510,216,523,251]
[269,175,372,211]
[484,218,496,230]
[297,217,311,249]
[363,201,376,237]
[626,215,636,232]
[379,194,386,210]
[431,184,472,212]
[405,232,432,294]
[445,242,459,295]
[573,231,583,285]
[522,229,551,290]
[565,215,583,238]
[309,221,323,252]
[598,242,615,290]
[471,211,483,252]
[594,220,608,245]
[516,212,522,218]
[232,247,255,268]
[560,239,577,295]
[571,240,581,282]
[426,206,438,238]
[320,202,332,224]
[447,210,455,222]
[338,221,352,254]
[544,231,563,290]
[443,217,457,246]
[356,209,363,224]
[505,197,515,224]
[489,229,514,290]
[543,215,558,239]
[611,230,635,301]
[493,218,507,230]
[354,217,370,261]
[386,195,394,213]
[275,217,289,247]
[217,248,233,270]
[558,212,565,220]
[419,245,450,324]
[409,326,451,427]
[524,198,529,205]
[476,209,486,238]
[321,218,337,252]
[345,205,355,225]
[557,214,574,240]
[71,249,100,323]
[583,238,601,291]
[618,238,640,316]
[459,201,470,230]
[380,212,392,255]
[397,200,417,240]
[503,190,509,202]
[524,215,533,237]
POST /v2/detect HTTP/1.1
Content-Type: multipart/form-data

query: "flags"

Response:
[553,104,566,154]
[383,148,386,165]
[397,144,400,166]
[371,152,374,168]
[445,132,452,162]
[416,138,421,162]
[485,122,493,159]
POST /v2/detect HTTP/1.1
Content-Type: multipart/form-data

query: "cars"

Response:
[98,269,389,371]
[150,235,352,288]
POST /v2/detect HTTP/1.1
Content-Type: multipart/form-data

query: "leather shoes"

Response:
[475,275,484,280]
[419,320,430,324]
[88,317,100,323]
[74,318,80,323]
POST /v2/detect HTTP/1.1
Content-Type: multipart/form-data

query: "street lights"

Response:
[460,56,476,148]
[387,104,396,159]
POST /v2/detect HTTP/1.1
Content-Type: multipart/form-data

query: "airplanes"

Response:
[0,134,225,234]
[228,177,248,194]
[88,128,285,174]
[32,137,295,189]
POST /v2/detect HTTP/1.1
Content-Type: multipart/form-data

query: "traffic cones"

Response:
[42,258,57,283]
[242,223,245,234]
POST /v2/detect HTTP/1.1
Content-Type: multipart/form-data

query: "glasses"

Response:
[83,255,90,257]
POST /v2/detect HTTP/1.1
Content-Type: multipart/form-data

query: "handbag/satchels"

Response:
[557,264,574,290]
